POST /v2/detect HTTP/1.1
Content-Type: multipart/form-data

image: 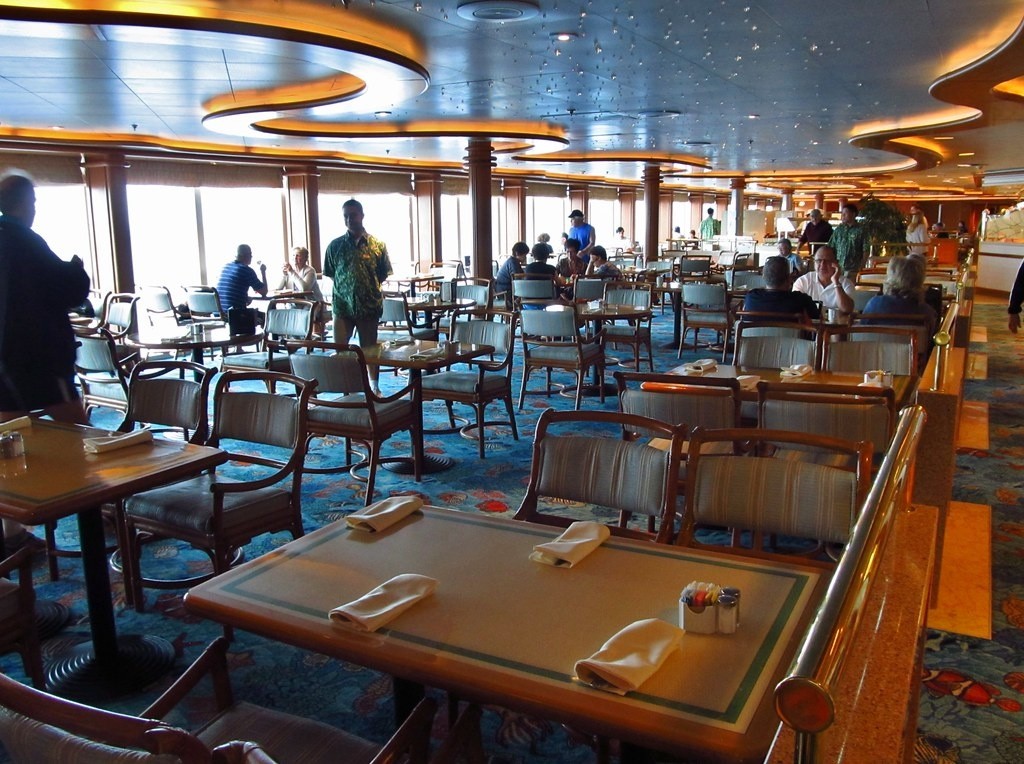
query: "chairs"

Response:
[60,239,962,650]
[626,282,694,350]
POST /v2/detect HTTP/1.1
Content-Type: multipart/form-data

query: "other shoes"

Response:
[2,528,47,557]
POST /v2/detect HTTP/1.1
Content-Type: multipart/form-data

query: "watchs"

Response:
[834,283,842,289]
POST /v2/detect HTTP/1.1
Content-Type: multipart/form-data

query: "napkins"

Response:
[410,348,444,360]
[735,375,770,392]
[855,382,885,399]
[0,414,684,694]
[779,365,813,378]
[388,335,415,345]
[185,321,226,328]
[685,359,716,373]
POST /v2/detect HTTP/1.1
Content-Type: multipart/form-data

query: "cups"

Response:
[828,308,839,323]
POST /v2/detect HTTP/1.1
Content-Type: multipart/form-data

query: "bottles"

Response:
[451,340,461,355]
[882,370,894,389]
[717,595,738,634]
[721,586,741,628]
[9,432,25,457]
[191,324,204,335]
[0,436,13,460]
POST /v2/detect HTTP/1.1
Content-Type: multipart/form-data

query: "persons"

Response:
[615,227,634,252]
[905,212,930,255]
[1007,258,1024,334]
[796,209,832,257]
[739,256,823,328]
[698,208,721,239]
[688,230,698,239]
[275,247,327,341]
[792,246,855,313]
[774,238,805,276]
[672,226,685,238]
[860,254,940,339]
[323,200,394,399]
[931,221,967,239]
[827,205,867,281]
[904,204,929,228]
[0,176,94,550]
[495,210,595,311]
[585,246,627,281]
[216,244,268,328]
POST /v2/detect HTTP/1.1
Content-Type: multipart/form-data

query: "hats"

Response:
[616,226,623,232]
[806,208,821,217]
[568,209,584,217]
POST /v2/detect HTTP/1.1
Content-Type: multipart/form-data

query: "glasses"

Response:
[813,258,834,264]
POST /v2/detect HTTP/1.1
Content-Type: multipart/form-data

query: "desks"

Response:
[384,274,444,325]
[182,495,835,763]
[249,289,293,352]
[122,321,266,440]
[391,297,477,328]
[325,332,495,475]
[0,410,228,708]
[621,268,670,282]
[494,303,653,400]
[665,362,917,427]
